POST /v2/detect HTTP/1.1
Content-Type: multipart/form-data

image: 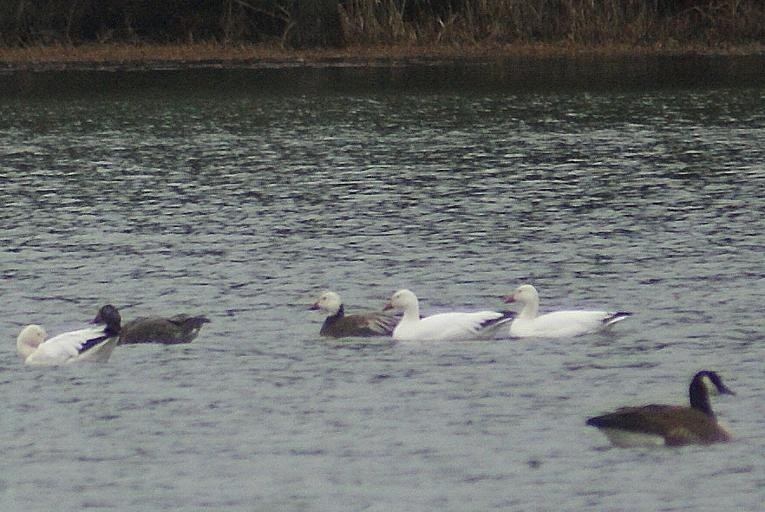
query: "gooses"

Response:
[14,322,122,368]
[582,370,739,449]
[382,288,517,342]
[87,304,213,345]
[304,291,424,338]
[503,281,634,341]
